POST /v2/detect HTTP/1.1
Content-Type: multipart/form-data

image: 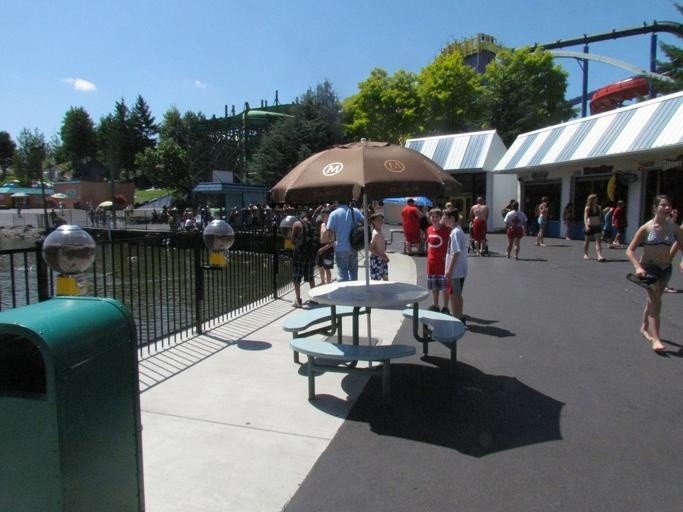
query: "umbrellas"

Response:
[268,136,465,370]
[98,200,113,208]
[50,192,69,199]
[10,191,29,198]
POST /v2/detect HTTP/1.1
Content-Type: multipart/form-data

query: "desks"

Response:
[310,280,429,369]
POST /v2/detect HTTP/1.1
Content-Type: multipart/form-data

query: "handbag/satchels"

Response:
[349,207,371,251]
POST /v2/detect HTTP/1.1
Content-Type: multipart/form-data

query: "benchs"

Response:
[289,337,416,400]
[283,307,366,362]
[403,309,465,375]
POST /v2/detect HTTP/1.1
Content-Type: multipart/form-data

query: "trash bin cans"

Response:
[0,294,145,512]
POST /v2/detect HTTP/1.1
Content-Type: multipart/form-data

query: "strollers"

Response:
[466,219,489,256]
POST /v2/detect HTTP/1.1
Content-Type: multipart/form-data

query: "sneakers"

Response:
[295,297,302,309]
[428,304,440,312]
[441,306,451,315]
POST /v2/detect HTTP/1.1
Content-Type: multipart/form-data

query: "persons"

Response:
[272,201,389,308]
[583,194,627,262]
[624,194,682,352]
[401,199,432,257]
[426,209,469,320]
[664,208,681,293]
[503,200,528,260]
[563,202,574,241]
[45,203,109,229]
[535,197,554,246]
[152,202,271,230]
[445,197,489,257]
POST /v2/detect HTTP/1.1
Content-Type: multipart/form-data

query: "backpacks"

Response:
[299,219,322,255]
